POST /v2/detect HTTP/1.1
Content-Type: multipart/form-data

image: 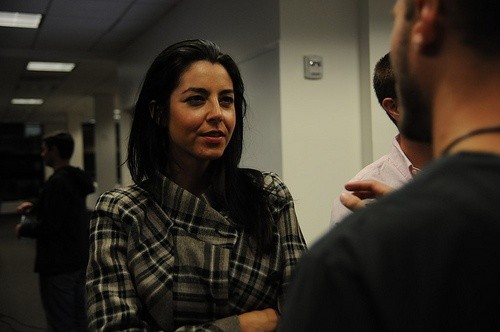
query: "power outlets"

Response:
[303,55,323,79]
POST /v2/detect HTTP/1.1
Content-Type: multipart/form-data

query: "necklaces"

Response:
[441,126,500,158]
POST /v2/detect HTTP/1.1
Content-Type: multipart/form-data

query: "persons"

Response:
[16,131,96,332]
[278,0,500,332]
[86,39,309,332]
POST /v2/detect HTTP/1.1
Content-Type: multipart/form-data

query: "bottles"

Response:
[12,210,30,253]
